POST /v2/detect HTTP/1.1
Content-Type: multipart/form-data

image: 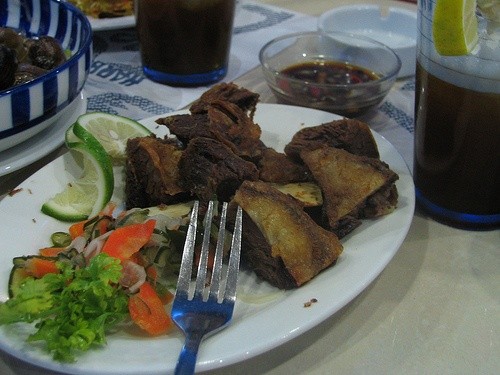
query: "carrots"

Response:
[27,203,174,336]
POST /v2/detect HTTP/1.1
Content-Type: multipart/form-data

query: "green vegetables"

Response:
[0,251,134,365]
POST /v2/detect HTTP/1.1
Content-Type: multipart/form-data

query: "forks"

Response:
[171,201,242,375]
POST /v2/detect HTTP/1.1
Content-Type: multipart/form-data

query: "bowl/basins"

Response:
[258,31,402,117]
[0,0,93,153]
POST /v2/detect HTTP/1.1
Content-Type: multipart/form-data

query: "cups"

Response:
[413,0,500,231]
[134,0,236,88]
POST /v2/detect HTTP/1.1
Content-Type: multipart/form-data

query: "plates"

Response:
[0,104,415,375]
[0,89,87,177]
[65,0,136,31]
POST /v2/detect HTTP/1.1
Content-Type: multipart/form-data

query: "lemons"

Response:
[433,0,481,56]
[40,110,158,220]
